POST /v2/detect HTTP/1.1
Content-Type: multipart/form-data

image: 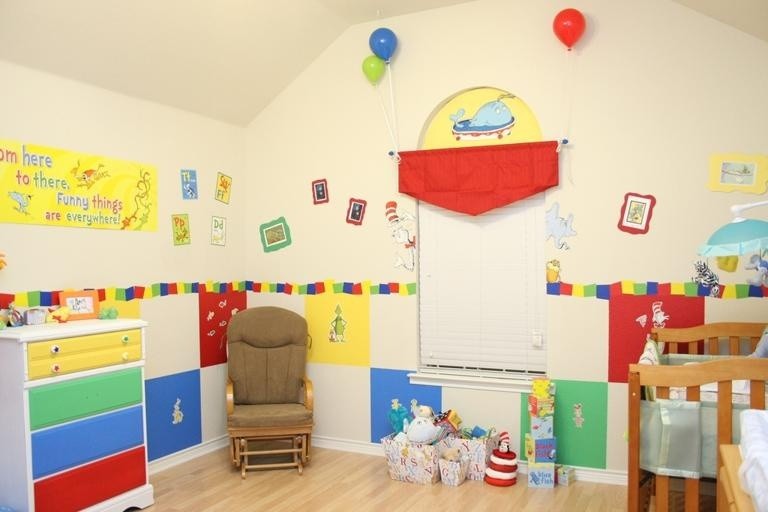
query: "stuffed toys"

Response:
[387,398,517,487]
[692,201,768,296]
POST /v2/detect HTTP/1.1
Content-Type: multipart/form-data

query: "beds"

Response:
[622,318,768,512]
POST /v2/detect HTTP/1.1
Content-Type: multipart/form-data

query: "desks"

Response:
[715,437,758,512]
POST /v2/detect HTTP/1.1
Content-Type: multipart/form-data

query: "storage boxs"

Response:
[379,394,577,488]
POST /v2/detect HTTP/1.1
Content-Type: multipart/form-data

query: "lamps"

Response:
[694,197,767,259]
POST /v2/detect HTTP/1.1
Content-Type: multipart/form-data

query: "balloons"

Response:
[369,28,397,64]
[553,9,585,50]
[362,55,385,86]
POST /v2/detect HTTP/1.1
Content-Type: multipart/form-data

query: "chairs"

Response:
[222,304,317,479]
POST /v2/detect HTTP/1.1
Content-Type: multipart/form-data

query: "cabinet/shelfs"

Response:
[0,317,158,511]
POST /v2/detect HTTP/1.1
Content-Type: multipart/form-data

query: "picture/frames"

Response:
[707,150,768,195]
[258,213,295,255]
[616,192,656,235]
[345,197,368,226]
[311,178,330,206]
